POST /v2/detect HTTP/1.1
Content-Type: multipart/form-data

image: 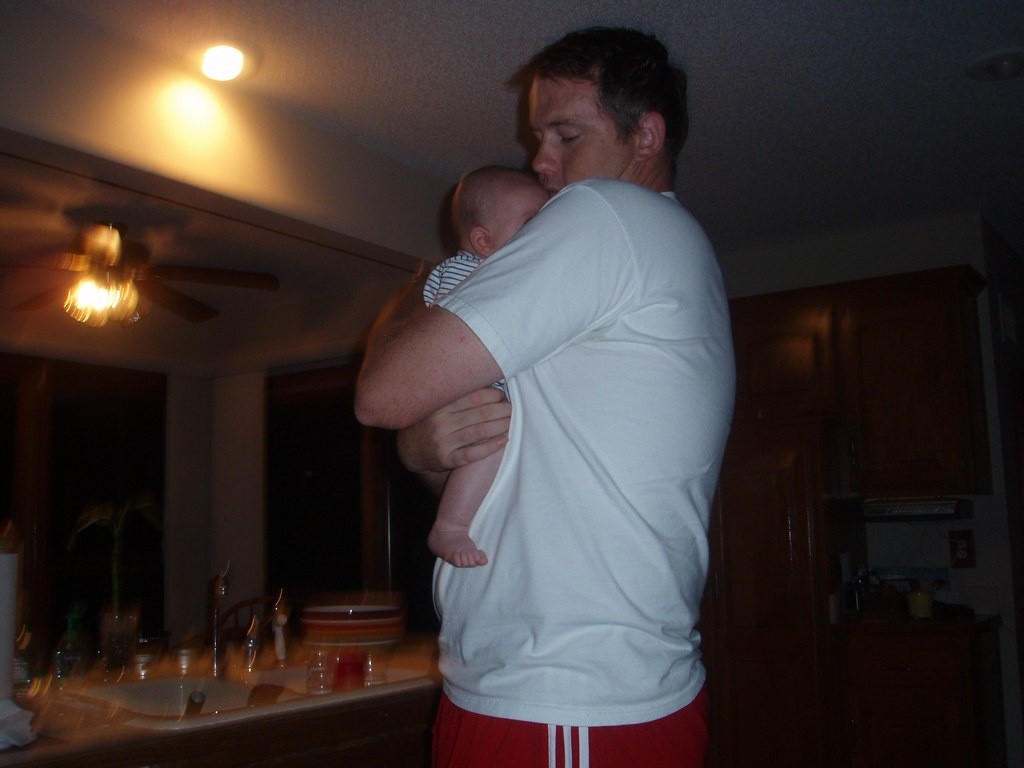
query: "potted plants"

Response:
[69,496,153,673]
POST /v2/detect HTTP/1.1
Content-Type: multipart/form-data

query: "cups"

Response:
[907,589,937,619]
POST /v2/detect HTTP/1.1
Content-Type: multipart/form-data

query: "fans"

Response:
[1,220,281,325]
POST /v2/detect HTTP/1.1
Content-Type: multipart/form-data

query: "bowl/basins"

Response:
[301,603,405,659]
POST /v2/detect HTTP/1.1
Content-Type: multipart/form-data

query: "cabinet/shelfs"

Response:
[699,282,1010,768]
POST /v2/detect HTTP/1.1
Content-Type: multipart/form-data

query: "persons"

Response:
[352,29,737,768]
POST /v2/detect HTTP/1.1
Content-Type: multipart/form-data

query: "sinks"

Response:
[65,670,267,731]
[238,653,435,705]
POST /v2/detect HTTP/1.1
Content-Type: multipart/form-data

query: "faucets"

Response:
[269,610,288,670]
[208,570,229,680]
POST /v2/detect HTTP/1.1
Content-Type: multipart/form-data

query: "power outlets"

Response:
[948,529,976,569]
